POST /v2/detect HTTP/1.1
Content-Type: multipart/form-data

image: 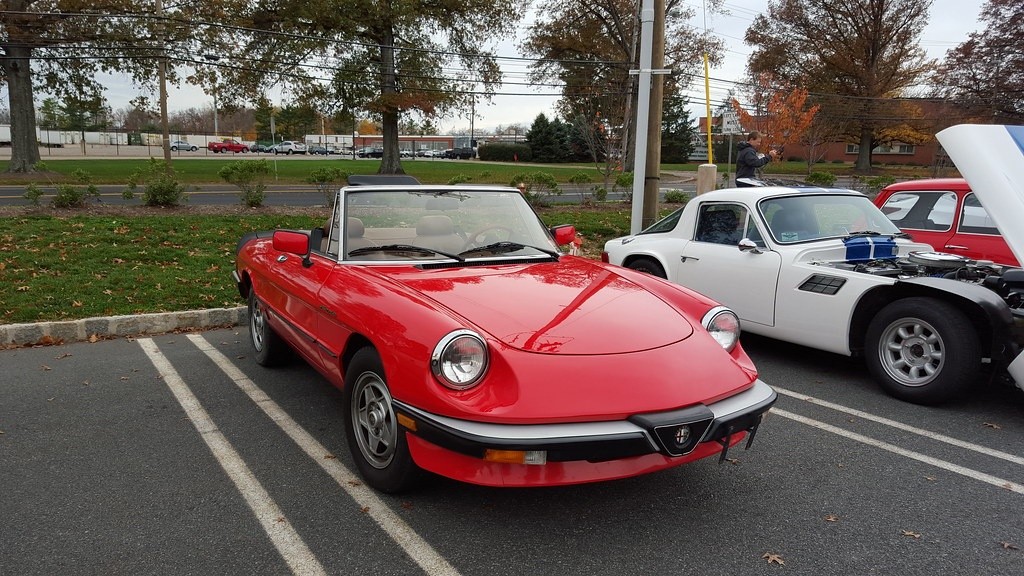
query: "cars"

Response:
[601,124,1024,408]
[870,176,1024,273]
[250,138,478,161]
[162,140,199,152]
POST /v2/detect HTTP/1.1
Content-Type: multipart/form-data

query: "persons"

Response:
[734,131,776,189]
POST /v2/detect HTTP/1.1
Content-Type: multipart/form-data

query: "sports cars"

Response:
[231,175,779,493]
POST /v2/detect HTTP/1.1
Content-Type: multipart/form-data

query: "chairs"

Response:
[771,208,819,240]
[699,209,737,245]
[411,215,465,258]
[320,216,383,259]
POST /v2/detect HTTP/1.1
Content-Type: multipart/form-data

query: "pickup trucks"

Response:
[207,138,250,154]
